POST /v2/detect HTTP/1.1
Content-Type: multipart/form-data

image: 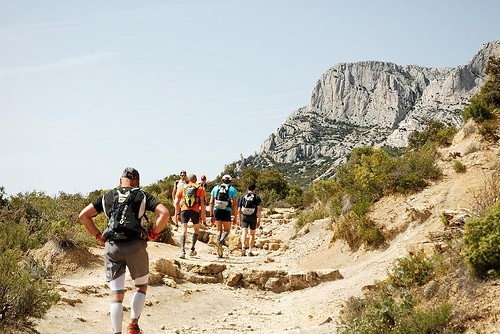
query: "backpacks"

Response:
[241,193,256,215]
[214,184,232,209]
[183,184,200,207]
[102,188,148,241]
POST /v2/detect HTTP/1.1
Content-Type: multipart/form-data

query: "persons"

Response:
[238,184,262,256]
[210,174,238,257]
[172,170,207,258]
[79,166,170,334]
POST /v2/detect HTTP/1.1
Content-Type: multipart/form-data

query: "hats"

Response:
[222,174,232,180]
[121,167,139,180]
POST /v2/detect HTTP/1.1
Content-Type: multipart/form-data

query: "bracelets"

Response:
[177,212,181,215]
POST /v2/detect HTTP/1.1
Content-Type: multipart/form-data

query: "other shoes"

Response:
[190,252,197,255]
[174,227,178,231]
[242,252,246,256]
[179,253,185,259]
[127,318,142,334]
[219,240,228,247]
[217,242,222,256]
[249,253,254,256]
